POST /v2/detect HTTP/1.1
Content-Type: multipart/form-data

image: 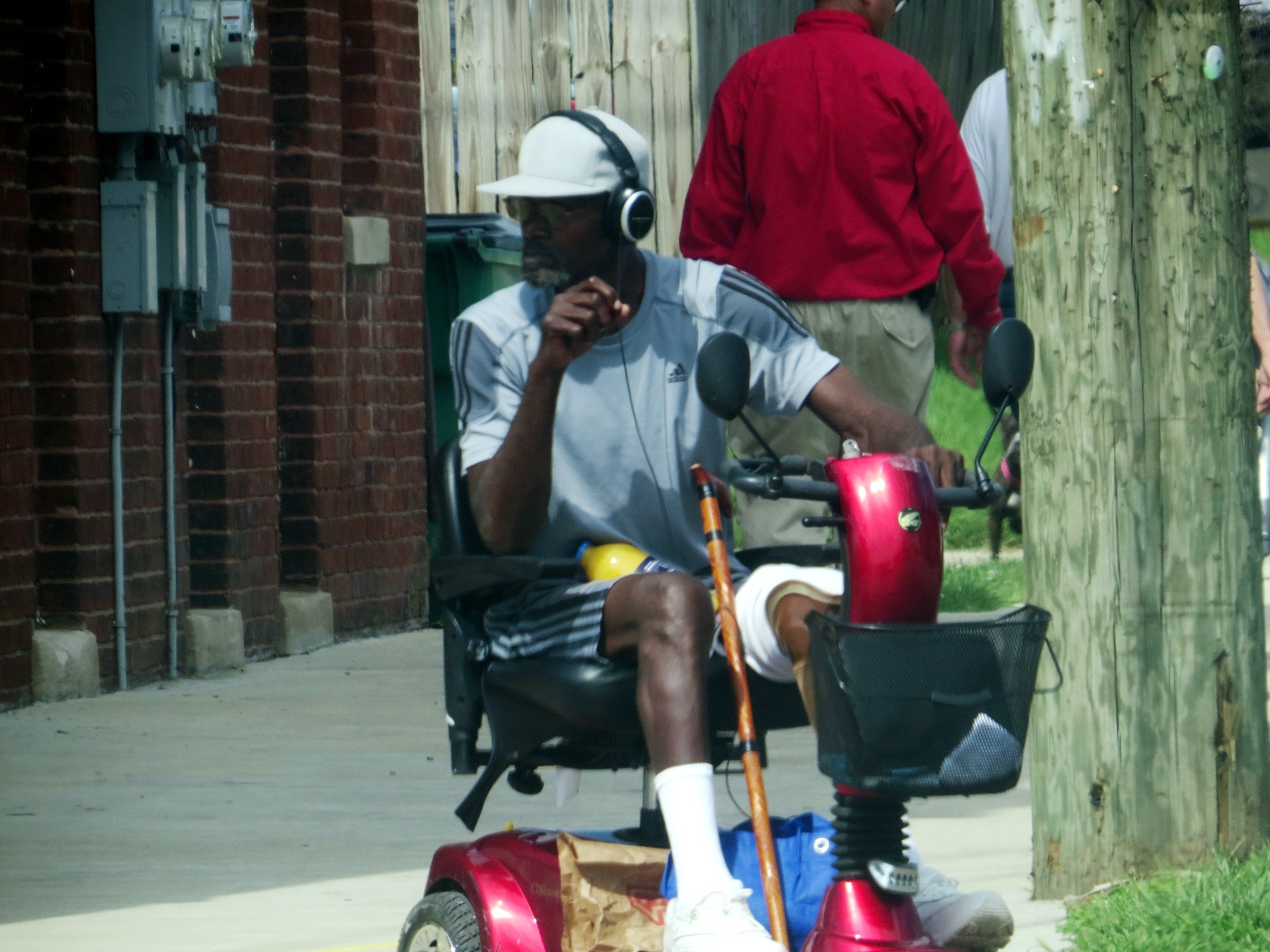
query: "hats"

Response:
[475,105,651,215]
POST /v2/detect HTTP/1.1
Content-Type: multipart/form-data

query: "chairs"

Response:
[420,437,811,848]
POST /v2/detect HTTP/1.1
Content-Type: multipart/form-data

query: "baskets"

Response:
[805,601,1049,794]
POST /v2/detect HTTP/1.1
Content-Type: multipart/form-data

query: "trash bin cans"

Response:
[419,211,527,629]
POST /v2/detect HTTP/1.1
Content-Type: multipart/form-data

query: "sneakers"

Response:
[662,879,789,952]
[901,854,1014,952]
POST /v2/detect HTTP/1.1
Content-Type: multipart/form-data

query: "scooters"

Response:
[392,316,1066,952]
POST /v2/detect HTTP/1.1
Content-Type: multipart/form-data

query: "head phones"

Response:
[536,109,658,247]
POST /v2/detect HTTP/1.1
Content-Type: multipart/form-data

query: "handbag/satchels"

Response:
[658,810,841,952]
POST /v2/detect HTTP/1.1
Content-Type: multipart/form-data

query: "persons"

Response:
[450,110,1014,952]
[949,67,1022,538]
[676,0,1010,560]
